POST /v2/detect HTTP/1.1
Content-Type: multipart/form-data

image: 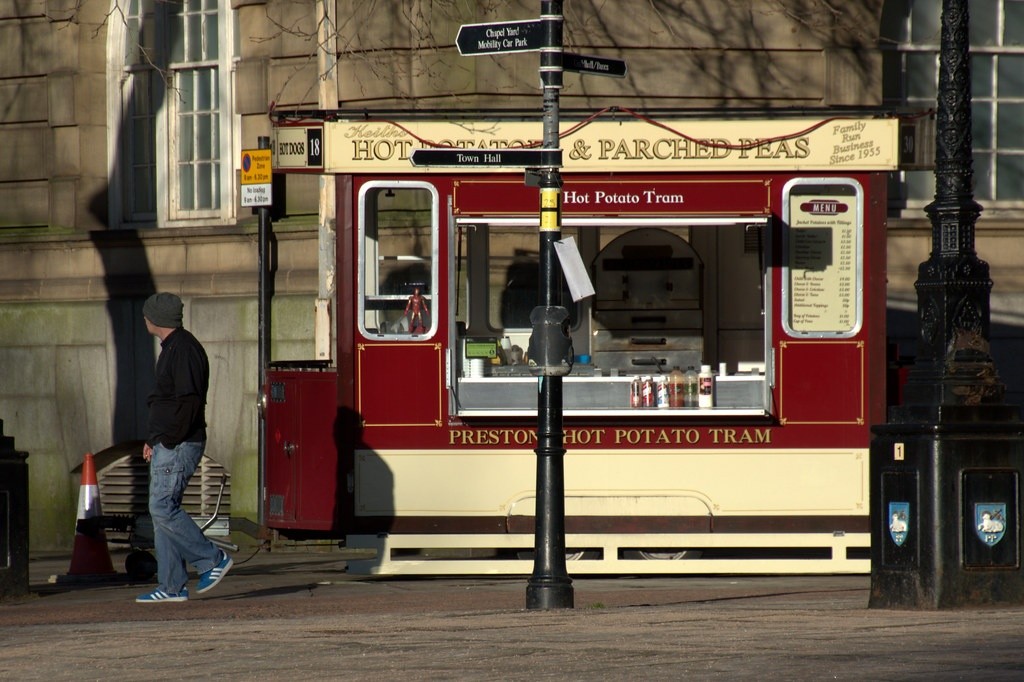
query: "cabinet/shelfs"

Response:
[590,228,704,372]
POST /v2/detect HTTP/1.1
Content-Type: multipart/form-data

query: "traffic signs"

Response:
[451,18,548,56]
[534,50,628,80]
[412,146,559,167]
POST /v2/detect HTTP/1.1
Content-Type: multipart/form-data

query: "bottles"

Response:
[642,376,656,407]
[656,375,669,407]
[669,366,684,407]
[697,365,713,407]
[682,365,698,407]
[630,375,642,407]
[501,336,512,365]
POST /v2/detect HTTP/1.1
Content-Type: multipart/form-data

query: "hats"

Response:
[143,292,184,328]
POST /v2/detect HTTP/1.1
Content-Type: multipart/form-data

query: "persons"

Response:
[135,292,233,602]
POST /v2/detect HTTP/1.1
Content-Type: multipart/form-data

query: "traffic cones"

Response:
[48,451,126,584]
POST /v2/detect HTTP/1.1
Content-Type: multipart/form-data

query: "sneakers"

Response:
[196,548,233,594]
[135,587,190,603]
